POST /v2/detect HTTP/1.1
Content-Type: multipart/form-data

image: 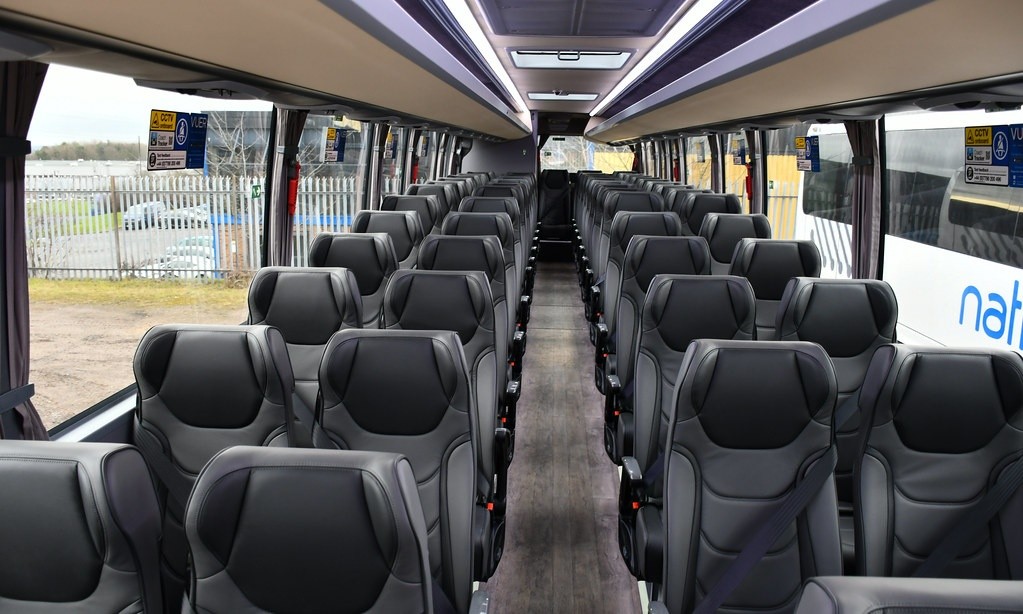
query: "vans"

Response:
[124,201,166,228]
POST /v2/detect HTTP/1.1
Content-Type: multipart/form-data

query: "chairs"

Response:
[0,168,1023,614]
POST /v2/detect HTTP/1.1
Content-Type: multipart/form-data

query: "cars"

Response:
[155,250,213,263]
[134,255,217,279]
[178,208,207,218]
[165,236,217,254]
[155,210,207,227]
